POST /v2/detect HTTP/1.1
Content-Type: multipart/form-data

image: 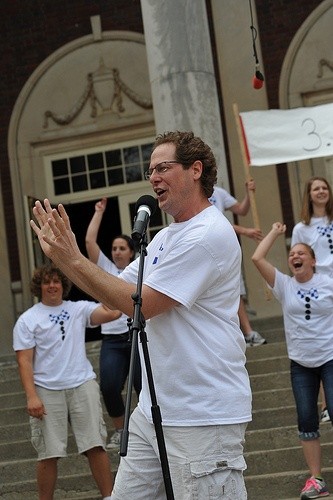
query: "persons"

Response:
[208,175,268,348]
[291,175,333,279]
[11,263,123,500]
[250,220,333,500]
[29,130,253,500]
[85,193,143,452]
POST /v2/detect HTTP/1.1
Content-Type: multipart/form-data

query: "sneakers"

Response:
[107,429,122,450]
[243,330,267,348]
[301,476,329,499]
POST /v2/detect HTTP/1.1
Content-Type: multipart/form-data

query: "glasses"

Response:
[144,160,190,180]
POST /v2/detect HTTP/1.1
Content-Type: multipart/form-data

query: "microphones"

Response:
[131,195,155,241]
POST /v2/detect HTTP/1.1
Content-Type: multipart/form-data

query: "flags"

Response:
[239,101,333,168]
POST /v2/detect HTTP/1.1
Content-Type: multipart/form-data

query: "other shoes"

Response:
[320,407,331,422]
